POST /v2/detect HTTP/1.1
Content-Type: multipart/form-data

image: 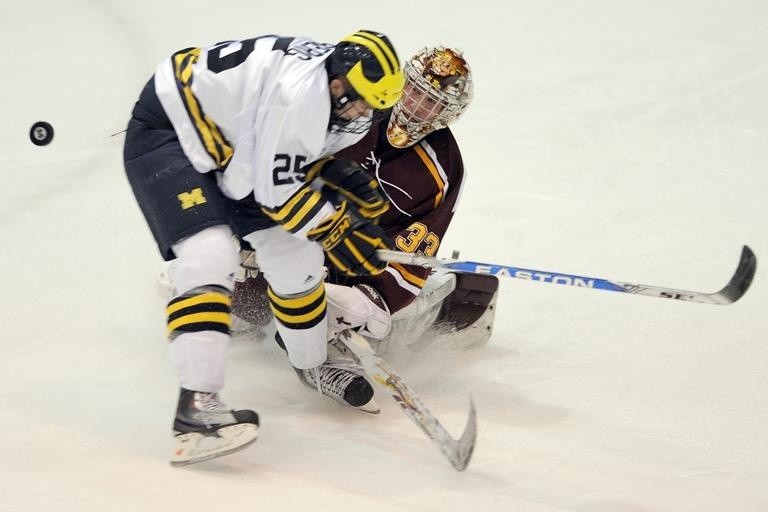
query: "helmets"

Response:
[326,29,404,133]
[385,47,474,148]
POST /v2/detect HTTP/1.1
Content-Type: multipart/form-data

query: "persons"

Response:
[121,27,405,465]
[156,45,496,365]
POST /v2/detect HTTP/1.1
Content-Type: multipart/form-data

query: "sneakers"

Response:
[174,388,258,432]
[293,358,374,407]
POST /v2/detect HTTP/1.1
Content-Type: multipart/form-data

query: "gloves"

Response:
[307,200,393,276]
[309,156,390,219]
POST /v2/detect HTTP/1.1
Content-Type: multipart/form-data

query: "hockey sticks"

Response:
[375,245,756,305]
[338,329,476,471]
